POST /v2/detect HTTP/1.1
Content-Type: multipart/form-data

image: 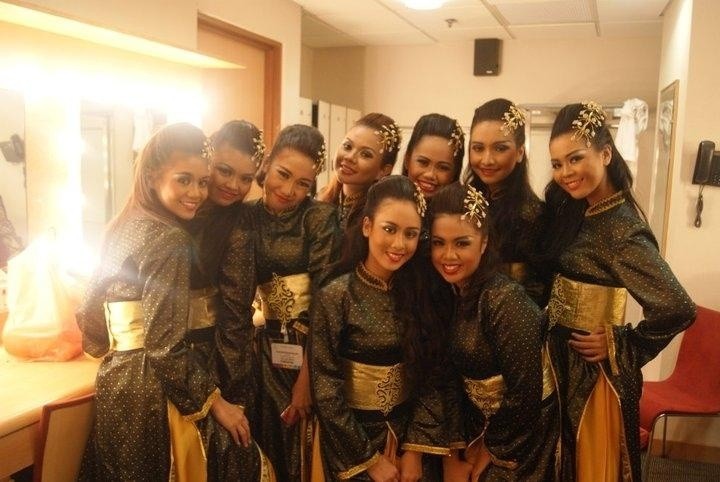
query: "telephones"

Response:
[692,140,720,187]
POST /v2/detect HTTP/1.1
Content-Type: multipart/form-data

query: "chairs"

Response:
[36,393,96,481]
[638,307,718,482]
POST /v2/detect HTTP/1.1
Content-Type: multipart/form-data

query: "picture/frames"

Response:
[649,79,678,263]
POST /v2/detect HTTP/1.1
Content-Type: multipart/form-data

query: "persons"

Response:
[461,97,547,285]
[397,113,467,200]
[423,180,566,482]
[238,124,345,482]
[302,174,449,482]
[72,122,254,481]
[535,99,700,482]
[315,113,402,270]
[187,118,268,482]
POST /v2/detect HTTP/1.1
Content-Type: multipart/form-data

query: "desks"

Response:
[0,344,110,481]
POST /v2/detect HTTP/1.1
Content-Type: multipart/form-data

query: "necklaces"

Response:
[335,186,369,207]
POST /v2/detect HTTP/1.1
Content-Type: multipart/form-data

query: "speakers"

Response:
[474,38,503,76]
